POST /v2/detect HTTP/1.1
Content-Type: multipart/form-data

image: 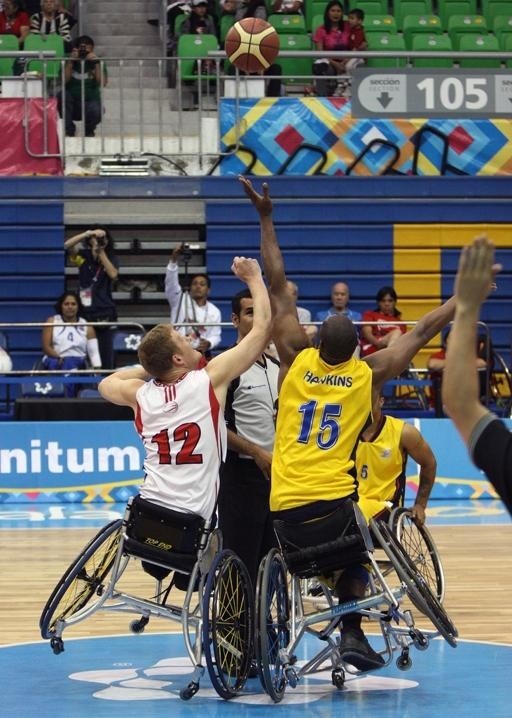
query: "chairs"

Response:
[0,0,79,95]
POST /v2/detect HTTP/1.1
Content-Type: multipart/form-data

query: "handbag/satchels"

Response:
[71,288,88,308]
[192,58,220,76]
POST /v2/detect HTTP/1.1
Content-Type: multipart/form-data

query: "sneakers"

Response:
[65,128,76,136]
[85,129,95,137]
[333,87,343,98]
[339,628,387,672]
[341,87,352,99]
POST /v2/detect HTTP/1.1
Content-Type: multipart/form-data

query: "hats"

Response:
[311,58,330,74]
[191,0,209,8]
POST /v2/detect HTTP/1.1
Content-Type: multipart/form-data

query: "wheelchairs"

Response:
[290,456,446,610]
[38,495,253,700]
[403,351,512,417]
[254,498,460,702]
[27,356,98,390]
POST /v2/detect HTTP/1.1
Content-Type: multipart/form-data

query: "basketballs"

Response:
[225,17,279,72]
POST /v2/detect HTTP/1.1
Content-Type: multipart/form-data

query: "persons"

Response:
[443,236,511,518]
[313,1,367,96]
[219,289,279,678]
[162,239,221,359]
[282,280,311,331]
[51,36,107,137]
[237,173,502,671]
[1,1,30,41]
[304,281,360,361]
[64,227,119,368]
[180,0,216,68]
[361,286,407,357]
[235,0,267,23]
[43,291,120,396]
[97,255,272,591]
[30,0,71,41]
[338,8,366,88]
[428,331,485,416]
[308,391,437,599]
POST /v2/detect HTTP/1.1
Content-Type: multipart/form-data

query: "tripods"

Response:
[173,262,201,337]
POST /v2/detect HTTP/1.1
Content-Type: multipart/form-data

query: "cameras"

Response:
[79,44,89,58]
[96,236,105,247]
[179,243,193,259]
